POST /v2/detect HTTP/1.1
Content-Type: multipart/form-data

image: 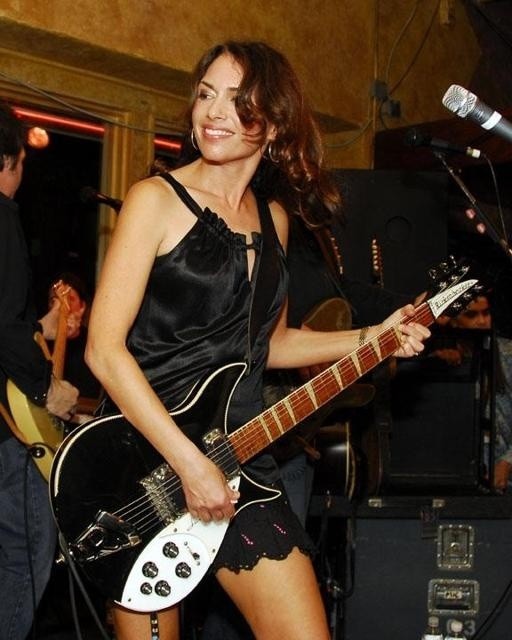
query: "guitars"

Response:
[6,280,72,484]
[49,252,488,614]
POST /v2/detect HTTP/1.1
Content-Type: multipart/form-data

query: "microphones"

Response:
[75,178,122,216]
[440,82,512,142]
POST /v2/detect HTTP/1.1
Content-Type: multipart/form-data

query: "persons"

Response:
[448,292,512,496]
[196,222,453,640]
[0,96,82,638]
[83,41,433,640]
[44,272,100,415]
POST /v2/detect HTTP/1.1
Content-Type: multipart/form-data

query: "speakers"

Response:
[341,498,512,639]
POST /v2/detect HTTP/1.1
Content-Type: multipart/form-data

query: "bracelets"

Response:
[359,324,367,347]
